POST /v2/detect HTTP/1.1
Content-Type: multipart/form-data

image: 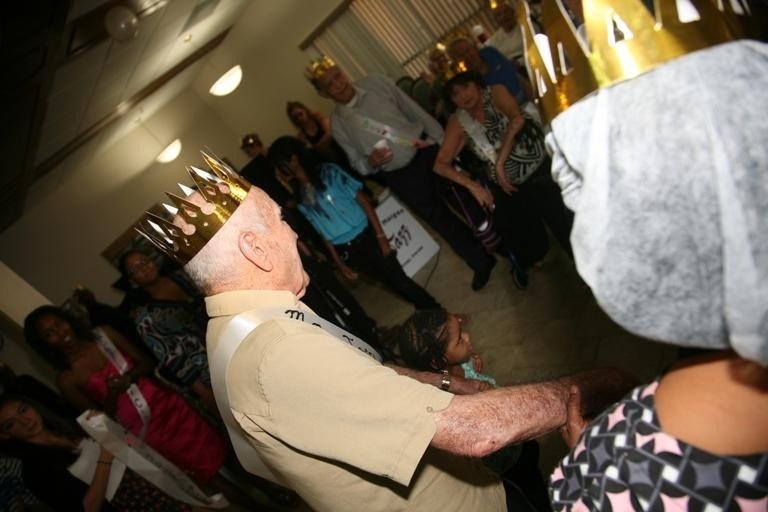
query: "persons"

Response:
[20,304,297,511]
[227,164,373,333]
[515,36,768,510]
[114,250,223,417]
[1,391,232,510]
[127,142,617,511]
[387,304,503,388]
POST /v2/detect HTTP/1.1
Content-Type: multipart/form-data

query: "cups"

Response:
[374,138,389,152]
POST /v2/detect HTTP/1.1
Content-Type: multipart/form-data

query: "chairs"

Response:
[411,77,436,114]
[396,76,415,96]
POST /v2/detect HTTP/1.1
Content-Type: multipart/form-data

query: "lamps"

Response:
[182,34,244,97]
[136,116,182,164]
[103,5,141,43]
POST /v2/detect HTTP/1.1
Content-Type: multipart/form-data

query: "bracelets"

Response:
[439,368,452,390]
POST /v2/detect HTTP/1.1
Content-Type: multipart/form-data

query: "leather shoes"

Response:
[472,255,498,290]
[512,265,528,290]
